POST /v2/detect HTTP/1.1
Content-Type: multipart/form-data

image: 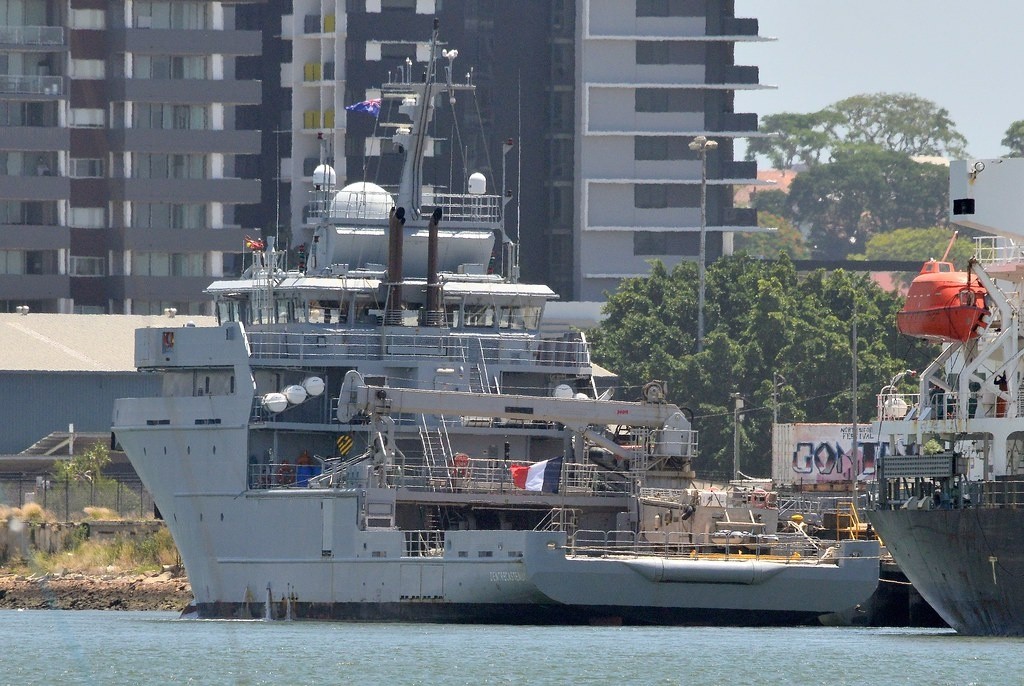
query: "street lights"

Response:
[688,135,719,353]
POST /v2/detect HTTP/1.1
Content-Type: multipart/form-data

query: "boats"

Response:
[862,156,1024,636]
[896,259,991,341]
[108,18,949,627]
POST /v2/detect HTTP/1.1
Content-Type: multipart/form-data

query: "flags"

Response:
[240,231,262,254]
[506,455,561,495]
[344,96,380,120]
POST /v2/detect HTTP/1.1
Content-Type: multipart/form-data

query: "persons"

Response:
[993,371,1007,417]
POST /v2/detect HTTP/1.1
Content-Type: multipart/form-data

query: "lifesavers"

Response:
[276,466,295,484]
[163,332,175,348]
[750,489,768,509]
